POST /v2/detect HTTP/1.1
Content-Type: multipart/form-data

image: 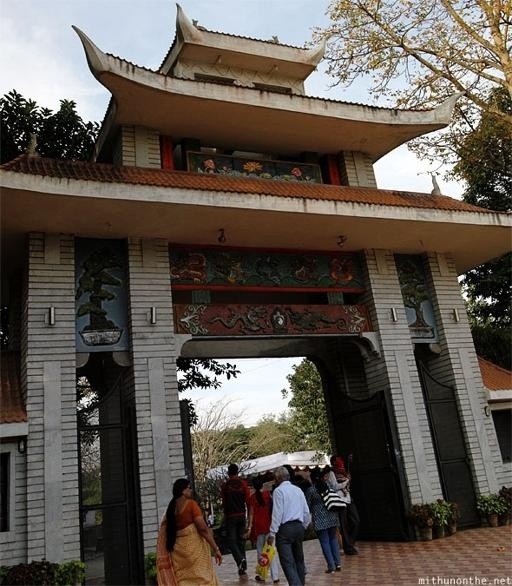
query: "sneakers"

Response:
[344,552,358,555]
[240,574,279,583]
[325,567,341,573]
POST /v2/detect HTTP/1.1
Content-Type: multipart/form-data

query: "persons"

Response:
[305,452,361,574]
[266,467,312,586]
[242,475,280,584]
[154,477,223,586]
[219,463,252,575]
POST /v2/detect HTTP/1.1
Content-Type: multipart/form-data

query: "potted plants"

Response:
[404,484,512,542]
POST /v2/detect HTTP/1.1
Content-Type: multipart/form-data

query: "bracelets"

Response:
[213,547,220,553]
[247,527,252,530]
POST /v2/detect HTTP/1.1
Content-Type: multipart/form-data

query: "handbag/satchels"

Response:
[321,489,346,512]
[255,541,276,578]
